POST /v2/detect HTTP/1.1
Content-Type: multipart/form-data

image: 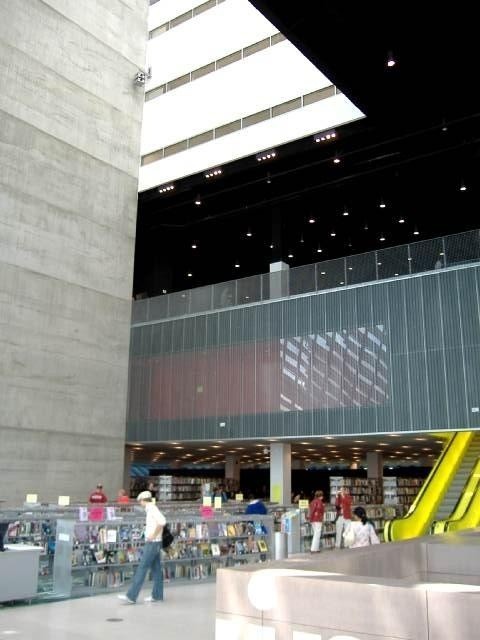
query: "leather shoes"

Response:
[117,593,135,604]
[142,596,162,604]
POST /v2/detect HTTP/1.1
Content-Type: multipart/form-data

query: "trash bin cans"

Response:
[275,532,288,560]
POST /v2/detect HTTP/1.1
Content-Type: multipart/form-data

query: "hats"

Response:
[136,490,153,501]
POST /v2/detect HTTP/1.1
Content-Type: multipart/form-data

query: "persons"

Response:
[309,490,324,554]
[116,491,174,603]
[113,489,128,502]
[146,482,158,497]
[245,495,267,534]
[335,487,353,550]
[89,484,107,503]
[342,507,380,548]
[213,484,243,502]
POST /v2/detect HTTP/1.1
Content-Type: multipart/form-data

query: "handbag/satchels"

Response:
[160,526,174,548]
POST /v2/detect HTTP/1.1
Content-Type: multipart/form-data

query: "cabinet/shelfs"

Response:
[7,503,404,597]
[327,474,427,513]
